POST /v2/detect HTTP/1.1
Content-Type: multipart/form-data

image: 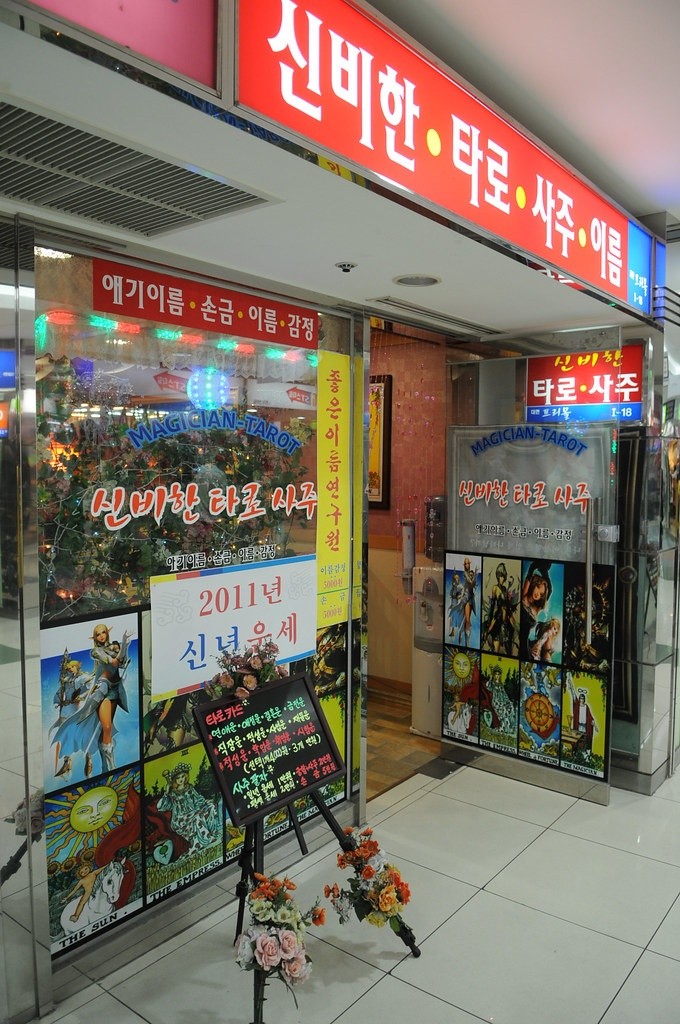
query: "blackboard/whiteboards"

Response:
[190,670,346,828]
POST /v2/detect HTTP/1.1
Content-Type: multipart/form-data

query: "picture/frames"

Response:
[369,374,390,510]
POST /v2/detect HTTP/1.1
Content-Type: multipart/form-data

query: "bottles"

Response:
[425,496,447,564]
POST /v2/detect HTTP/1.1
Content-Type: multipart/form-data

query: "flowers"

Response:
[205,638,288,700]
[233,871,325,1024]
[323,826,416,947]
